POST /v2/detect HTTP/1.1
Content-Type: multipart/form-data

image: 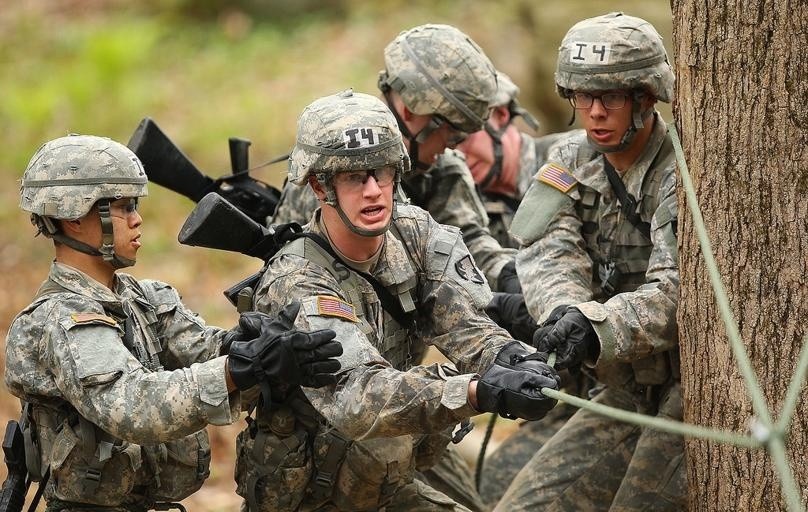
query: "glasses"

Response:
[439,127,470,147]
[567,92,636,111]
[332,168,398,188]
[109,199,140,220]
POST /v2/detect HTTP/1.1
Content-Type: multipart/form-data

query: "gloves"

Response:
[497,262,523,294]
[476,305,592,422]
[484,293,542,347]
[228,302,344,413]
[220,302,274,355]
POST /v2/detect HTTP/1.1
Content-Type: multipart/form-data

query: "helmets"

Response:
[554,13,677,105]
[19,135,149,222]
[491,70,520,109]
[384,25,500,135]
[288,93,413,189]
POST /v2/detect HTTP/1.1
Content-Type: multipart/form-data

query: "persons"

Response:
[5,136,342,511]
[237,13,687,512]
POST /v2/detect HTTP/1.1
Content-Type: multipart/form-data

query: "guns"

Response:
[179,193,304,314]
[1,420,33,510]
[126,118,284,227]
